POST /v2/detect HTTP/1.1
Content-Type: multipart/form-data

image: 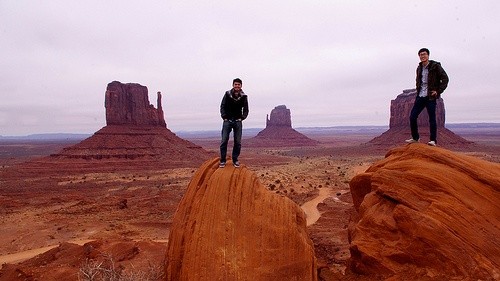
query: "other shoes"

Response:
[428,141,436,147]
[233,161,240,168]
[406,139,419,144]
[219,162,226,168]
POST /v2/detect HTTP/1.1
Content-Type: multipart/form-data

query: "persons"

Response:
[405,48,449,146]
[218,78,249,168]
